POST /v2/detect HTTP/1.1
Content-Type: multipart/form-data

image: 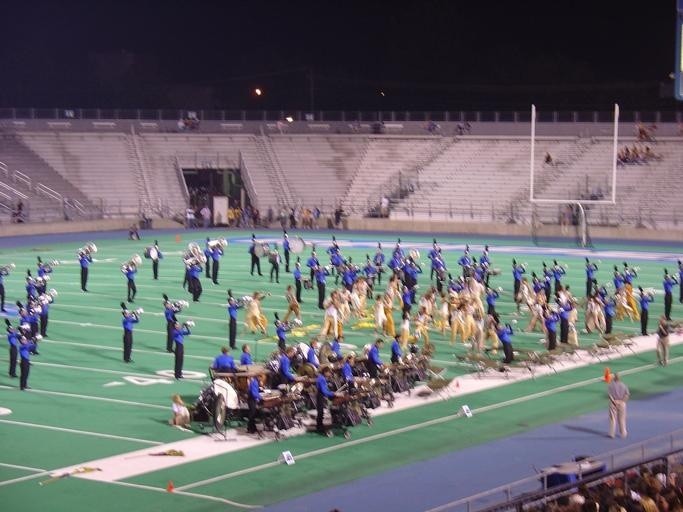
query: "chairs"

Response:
[423,331,639,404]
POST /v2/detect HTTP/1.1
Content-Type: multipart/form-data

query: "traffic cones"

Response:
[604,366,609,382]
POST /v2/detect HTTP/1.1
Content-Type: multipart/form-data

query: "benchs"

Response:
[0,132,683,230]
